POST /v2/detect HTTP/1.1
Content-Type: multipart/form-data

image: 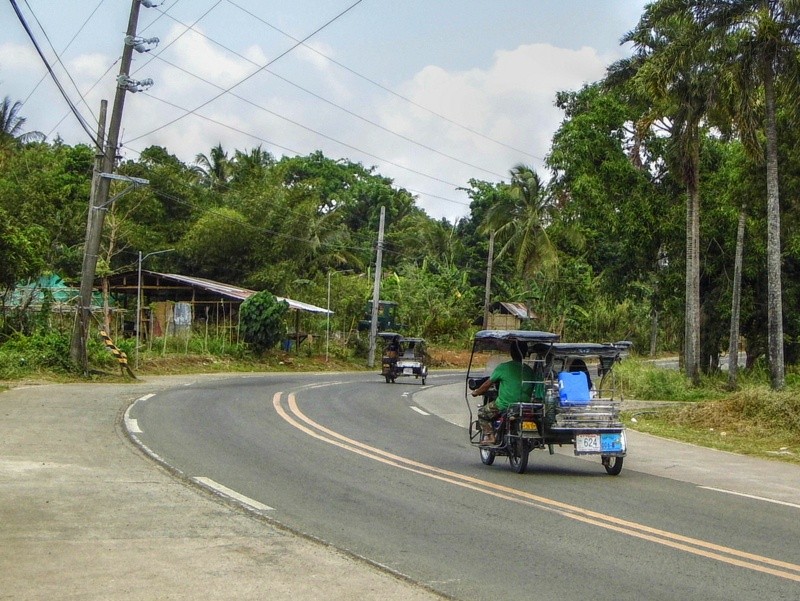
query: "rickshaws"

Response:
[377,333,428,385]
[466,330,632,475]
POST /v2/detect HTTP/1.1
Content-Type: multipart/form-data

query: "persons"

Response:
[471,341,534,443]
[569,359,597,398]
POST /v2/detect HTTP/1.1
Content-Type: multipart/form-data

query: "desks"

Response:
[287,334,321,356]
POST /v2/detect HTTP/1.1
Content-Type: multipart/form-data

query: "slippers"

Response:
[480,439,495,446]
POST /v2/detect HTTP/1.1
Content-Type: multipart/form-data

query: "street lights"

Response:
[135,249,174,368]
[326,270,352,359]
